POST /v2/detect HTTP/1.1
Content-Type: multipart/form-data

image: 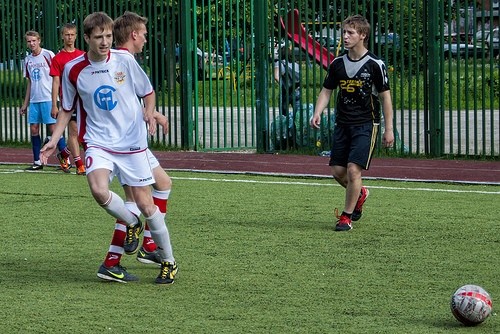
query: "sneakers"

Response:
[124,214,143,254]
[352,187,369,221]
[25,164,44,171]
[97,263,139,283]
[334,208,353,231]
[155,261,179,285]
[68,163,73,169]
[136,245,163,265]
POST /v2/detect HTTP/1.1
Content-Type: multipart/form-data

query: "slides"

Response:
[278,7,336,72]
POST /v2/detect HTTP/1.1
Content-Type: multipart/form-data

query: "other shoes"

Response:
[57,153,70,173]
[76,165,86,175]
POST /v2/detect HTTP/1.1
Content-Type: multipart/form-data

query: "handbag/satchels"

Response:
[281,67,300,95]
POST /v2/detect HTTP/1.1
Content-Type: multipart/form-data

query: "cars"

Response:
[146,28,500,79]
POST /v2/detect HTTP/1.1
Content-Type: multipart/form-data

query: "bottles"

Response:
[319,151,331,157]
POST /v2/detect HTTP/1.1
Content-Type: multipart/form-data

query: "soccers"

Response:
[450,284,493,326]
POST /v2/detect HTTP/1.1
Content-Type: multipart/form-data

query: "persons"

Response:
[40,12,179,285]
[312,15,395,230]
[275,46,300,116]
[76,12,172,283]
[19,31,72,172]
[49,24,86,174]
